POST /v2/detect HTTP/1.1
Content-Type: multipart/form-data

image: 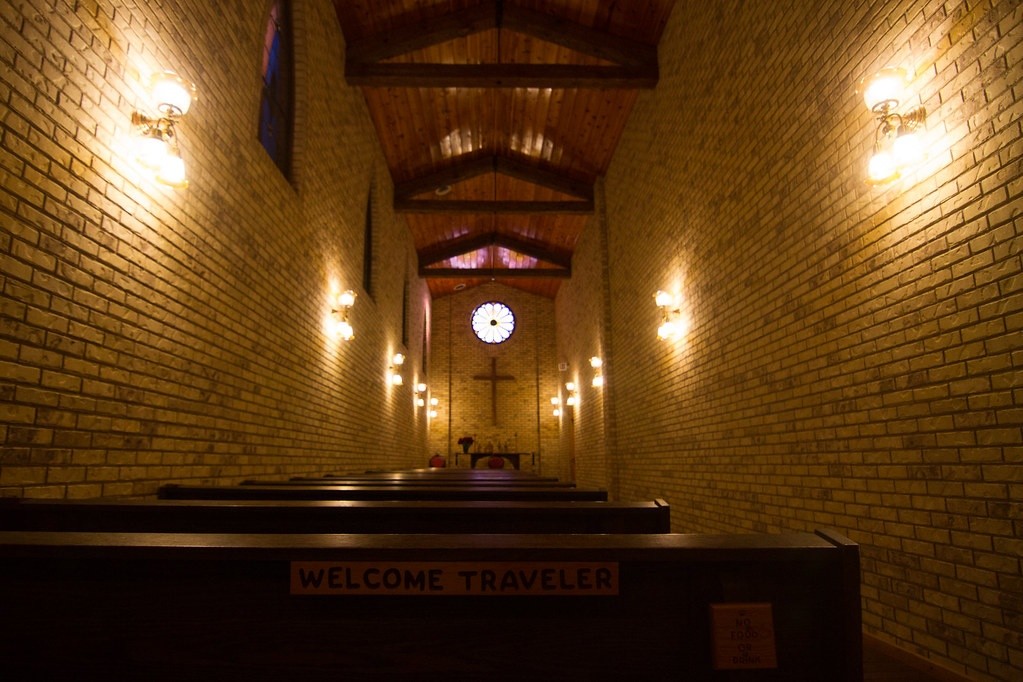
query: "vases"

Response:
[463,446,468,453]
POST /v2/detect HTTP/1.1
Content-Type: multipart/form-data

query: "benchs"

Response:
[0,469,868,682]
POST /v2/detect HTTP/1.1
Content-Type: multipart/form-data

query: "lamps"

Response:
[132,70,197,191]
[565,382,575,406]
[550,397,560,417]
[389,354,405,386]
[651,289,681,341]
[331,290,357,341]
[588,357,602,388]
[415,383,427,408]
[430,398,439,417]
[860,67,926,186]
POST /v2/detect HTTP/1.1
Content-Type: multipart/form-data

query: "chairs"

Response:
[488,456,505,469]
[429,454,446,468]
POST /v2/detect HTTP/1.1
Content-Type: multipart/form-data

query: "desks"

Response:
[458,453,530,470]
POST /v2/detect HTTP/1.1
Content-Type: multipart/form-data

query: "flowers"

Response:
[457,436,473,451]
